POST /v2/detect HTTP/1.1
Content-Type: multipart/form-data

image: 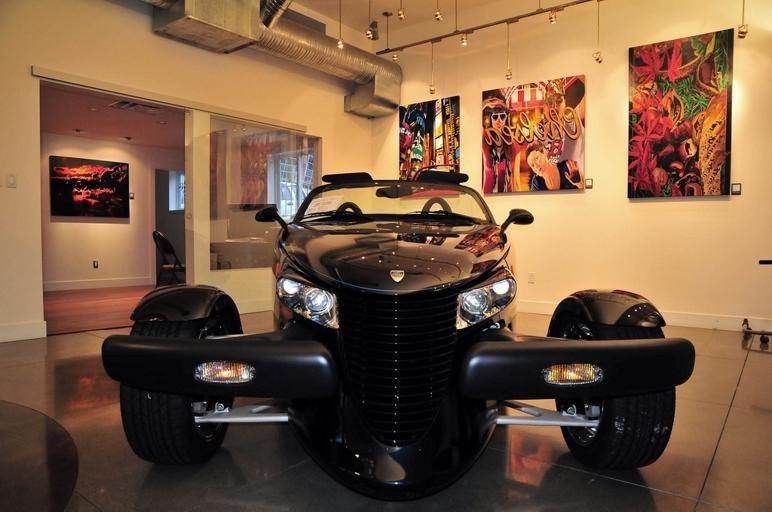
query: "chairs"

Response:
[422,197,451,214]
[337,201,362,216]
[152,230,186,288]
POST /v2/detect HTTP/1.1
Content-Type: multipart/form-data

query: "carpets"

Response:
[0,398,79,512]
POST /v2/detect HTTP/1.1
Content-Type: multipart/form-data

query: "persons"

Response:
[523,140,584,191]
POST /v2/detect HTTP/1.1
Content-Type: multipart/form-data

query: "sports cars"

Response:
[103,169,694,502]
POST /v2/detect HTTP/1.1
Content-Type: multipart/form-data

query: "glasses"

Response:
[491,111,507,121]
